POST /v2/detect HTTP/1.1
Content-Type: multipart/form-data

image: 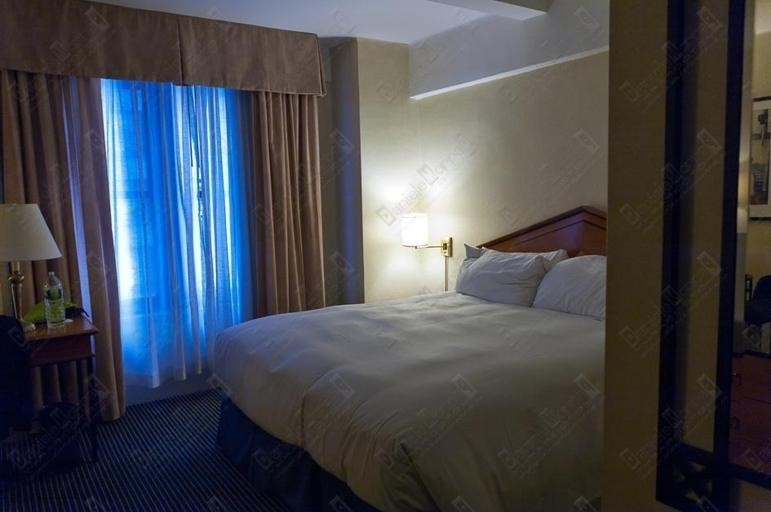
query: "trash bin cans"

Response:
[39,402,81,464]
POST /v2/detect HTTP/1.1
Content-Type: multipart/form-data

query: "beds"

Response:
[213,206,608,512]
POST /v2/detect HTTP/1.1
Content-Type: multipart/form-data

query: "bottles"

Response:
[42,271,66,328]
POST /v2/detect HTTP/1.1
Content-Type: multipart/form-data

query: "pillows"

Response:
[455,243,607,321]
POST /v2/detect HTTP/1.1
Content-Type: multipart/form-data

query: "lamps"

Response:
[400,213,453,260]
[0,203,63,332]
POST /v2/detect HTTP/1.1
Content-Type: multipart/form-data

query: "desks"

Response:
[23,307,99,436]
